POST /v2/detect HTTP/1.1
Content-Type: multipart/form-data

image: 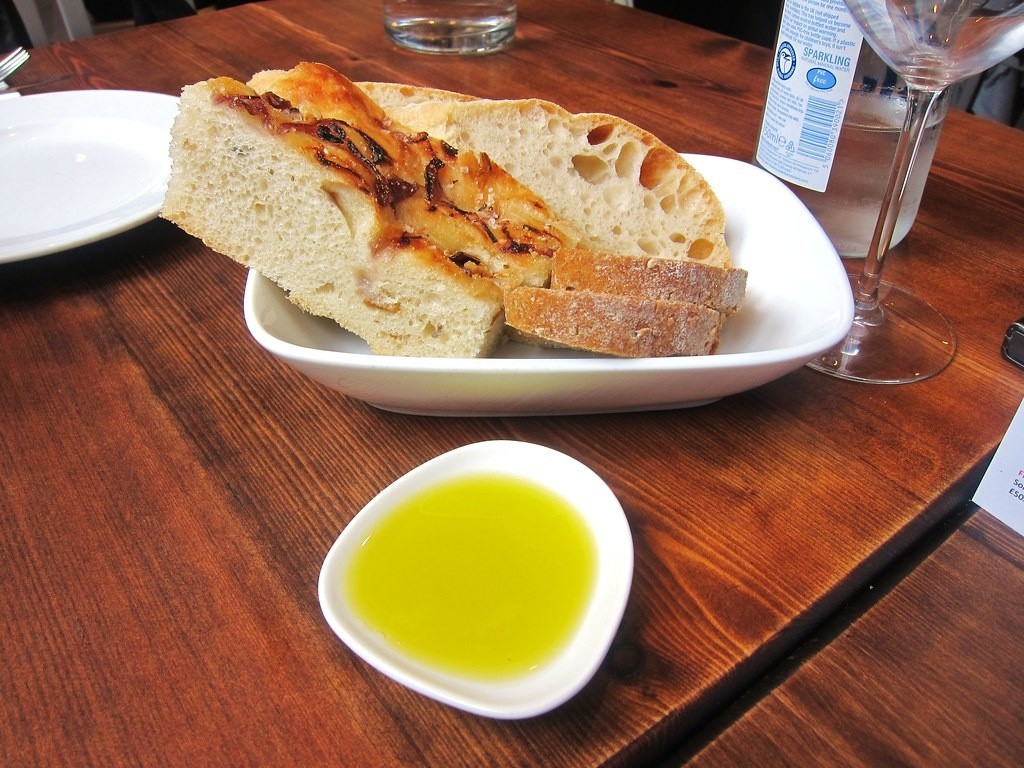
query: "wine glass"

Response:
[806,0,1024,384]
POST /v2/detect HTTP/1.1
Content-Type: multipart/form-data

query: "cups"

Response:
[383,0,518,56]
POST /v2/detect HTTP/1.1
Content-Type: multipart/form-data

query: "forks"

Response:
[0,47,30,81]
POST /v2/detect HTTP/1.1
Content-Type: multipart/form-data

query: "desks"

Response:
[682,509,1024,767]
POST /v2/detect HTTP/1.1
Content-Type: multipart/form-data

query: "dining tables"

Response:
[2,1,1023,766]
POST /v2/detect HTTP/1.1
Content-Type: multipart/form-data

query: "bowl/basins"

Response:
[243,153,855,416]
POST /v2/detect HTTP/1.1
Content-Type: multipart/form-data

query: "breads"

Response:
[156,62,747,359]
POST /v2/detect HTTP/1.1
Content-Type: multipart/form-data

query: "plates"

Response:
[0,89,182,263]
[316,440,636,722]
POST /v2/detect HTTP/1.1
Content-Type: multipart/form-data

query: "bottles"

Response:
[753,0,963,258]
